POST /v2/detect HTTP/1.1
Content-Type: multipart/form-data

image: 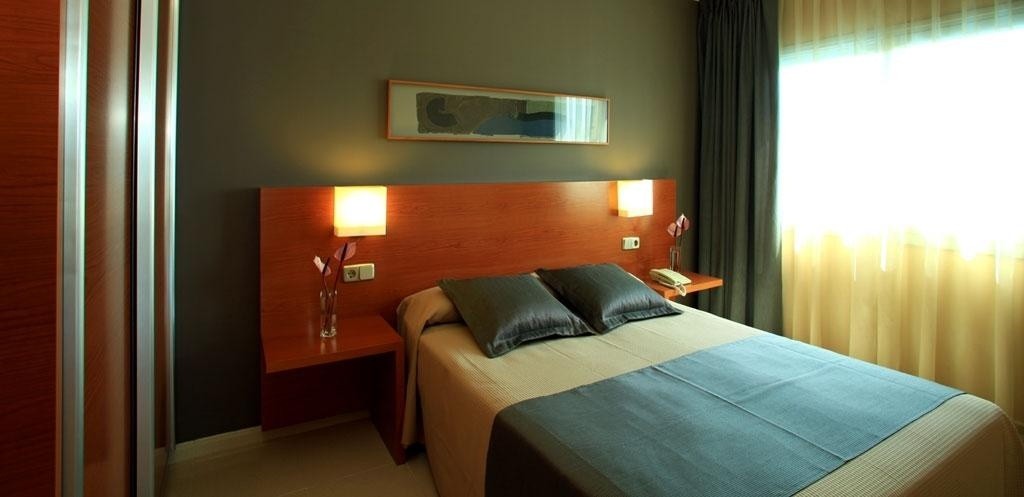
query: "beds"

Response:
[401,262,1009,495]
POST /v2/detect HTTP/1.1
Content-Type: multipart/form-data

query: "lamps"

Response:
[615,178,656,218]
[331,183,388,241]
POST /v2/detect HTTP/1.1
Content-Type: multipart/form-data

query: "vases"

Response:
[319,288,339,337]
[668,245,681,272]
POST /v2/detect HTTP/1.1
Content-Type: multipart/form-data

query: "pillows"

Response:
[434,263,684,359]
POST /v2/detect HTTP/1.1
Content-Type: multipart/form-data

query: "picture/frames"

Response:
[385,75,610,147]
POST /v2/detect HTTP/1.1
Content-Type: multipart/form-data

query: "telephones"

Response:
[648,267,692,288]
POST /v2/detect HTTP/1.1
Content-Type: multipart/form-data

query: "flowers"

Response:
[311,240,356,334]
[666,210,690,270]
